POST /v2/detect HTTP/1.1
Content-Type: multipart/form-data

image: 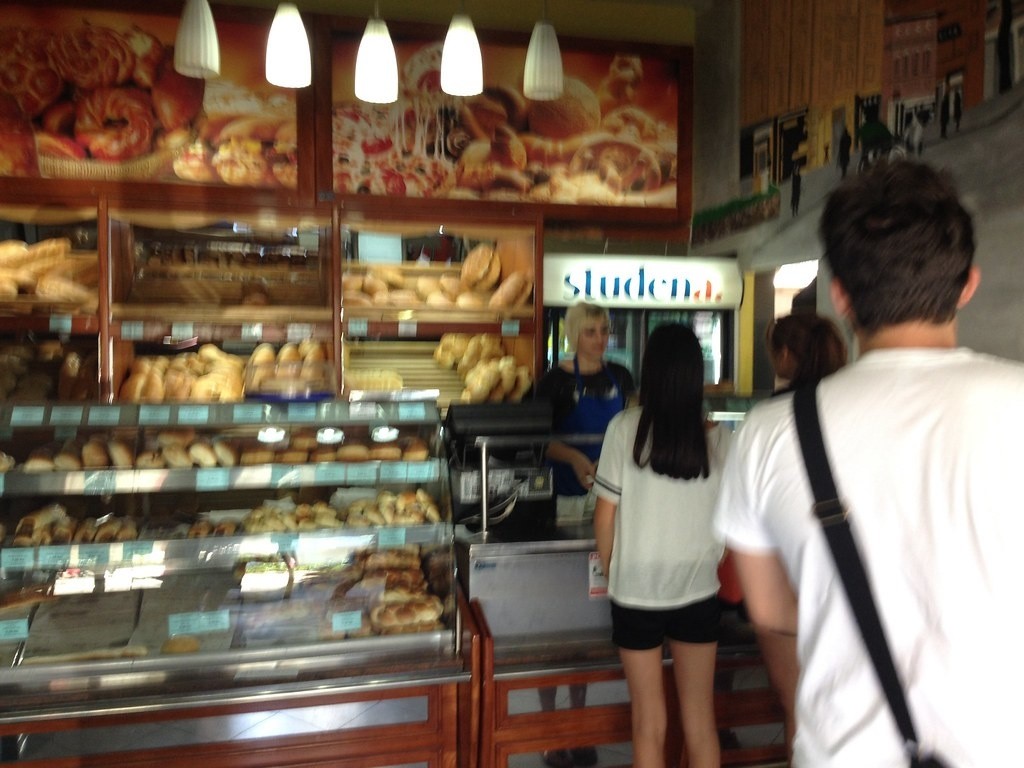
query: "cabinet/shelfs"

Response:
[0,193,542,768]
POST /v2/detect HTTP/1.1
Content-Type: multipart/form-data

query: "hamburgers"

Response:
[174,114,300,189]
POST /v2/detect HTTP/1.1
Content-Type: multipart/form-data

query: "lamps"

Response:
[355,0,399,104]
[440,0,483,96]
[524,0,563,101]
[174,1,220,79]
[266,1,312,89]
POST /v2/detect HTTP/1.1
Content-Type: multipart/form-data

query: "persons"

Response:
[766,313,848,399]
[712,158,1023,768]
[531,302,635,496]
[591,319,736,768]
[536,681,598,768]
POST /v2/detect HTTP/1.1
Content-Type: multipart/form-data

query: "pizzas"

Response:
[399,40,462,107]
[331,99,456,198]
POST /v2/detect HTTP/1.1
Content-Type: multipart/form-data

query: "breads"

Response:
[438,55,679,205]
[0,237,532,631]
[0,18,206,176]
